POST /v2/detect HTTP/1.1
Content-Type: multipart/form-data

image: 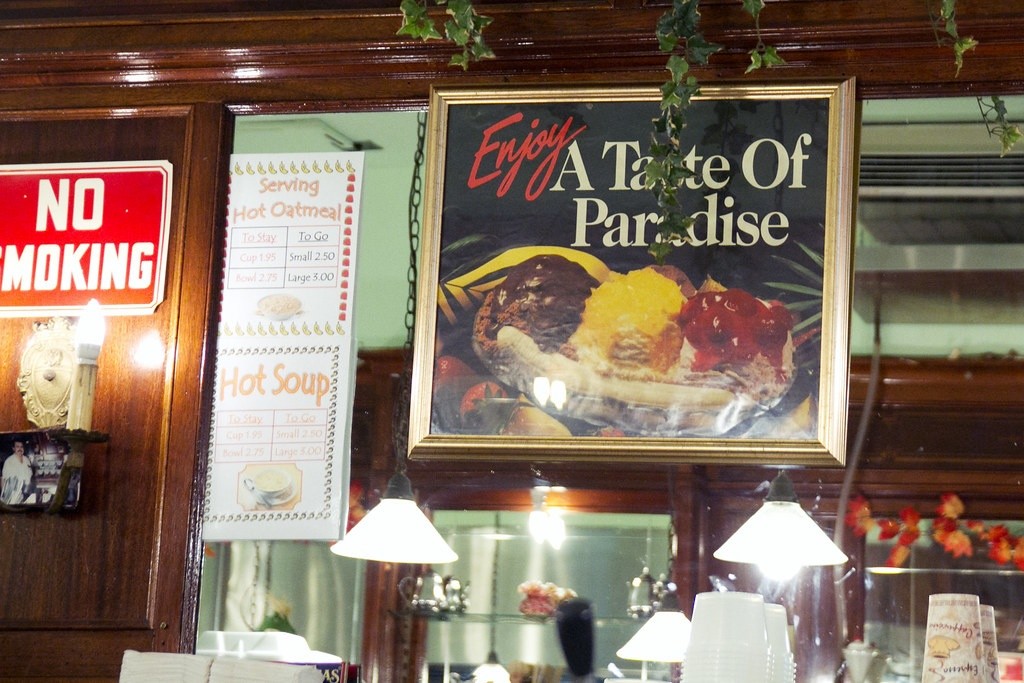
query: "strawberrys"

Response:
[436,355,510,423]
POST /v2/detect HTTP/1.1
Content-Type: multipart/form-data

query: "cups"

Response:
[921,593,1001,683]
[841,648,889,683]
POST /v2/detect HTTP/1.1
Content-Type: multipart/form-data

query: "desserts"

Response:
[472,256,796,434]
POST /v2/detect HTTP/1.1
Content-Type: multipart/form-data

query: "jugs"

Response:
[442,576,473,613]
[626,567,662,619]
[397,567,447,613]
[654,574,679,610]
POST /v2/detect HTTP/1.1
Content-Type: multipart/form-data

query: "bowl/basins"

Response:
[681,591,797,683]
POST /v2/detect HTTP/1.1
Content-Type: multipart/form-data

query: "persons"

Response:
[2,440,33,504]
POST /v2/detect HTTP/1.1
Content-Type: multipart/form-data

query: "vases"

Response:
[519,595,554,618]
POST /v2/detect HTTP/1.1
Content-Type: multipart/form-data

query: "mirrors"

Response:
[396,487,698,683]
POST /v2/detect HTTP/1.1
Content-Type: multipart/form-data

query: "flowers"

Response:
[516,578,579,603]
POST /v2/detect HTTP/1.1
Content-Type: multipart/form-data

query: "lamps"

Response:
[473,511,512,683]
[65,299,108,431]
[330,109,460,566]
[711,468,849,569]
[615,587,695,663]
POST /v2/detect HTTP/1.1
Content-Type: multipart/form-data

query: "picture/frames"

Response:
[0,432,85,513]
[406,74,860,467]
